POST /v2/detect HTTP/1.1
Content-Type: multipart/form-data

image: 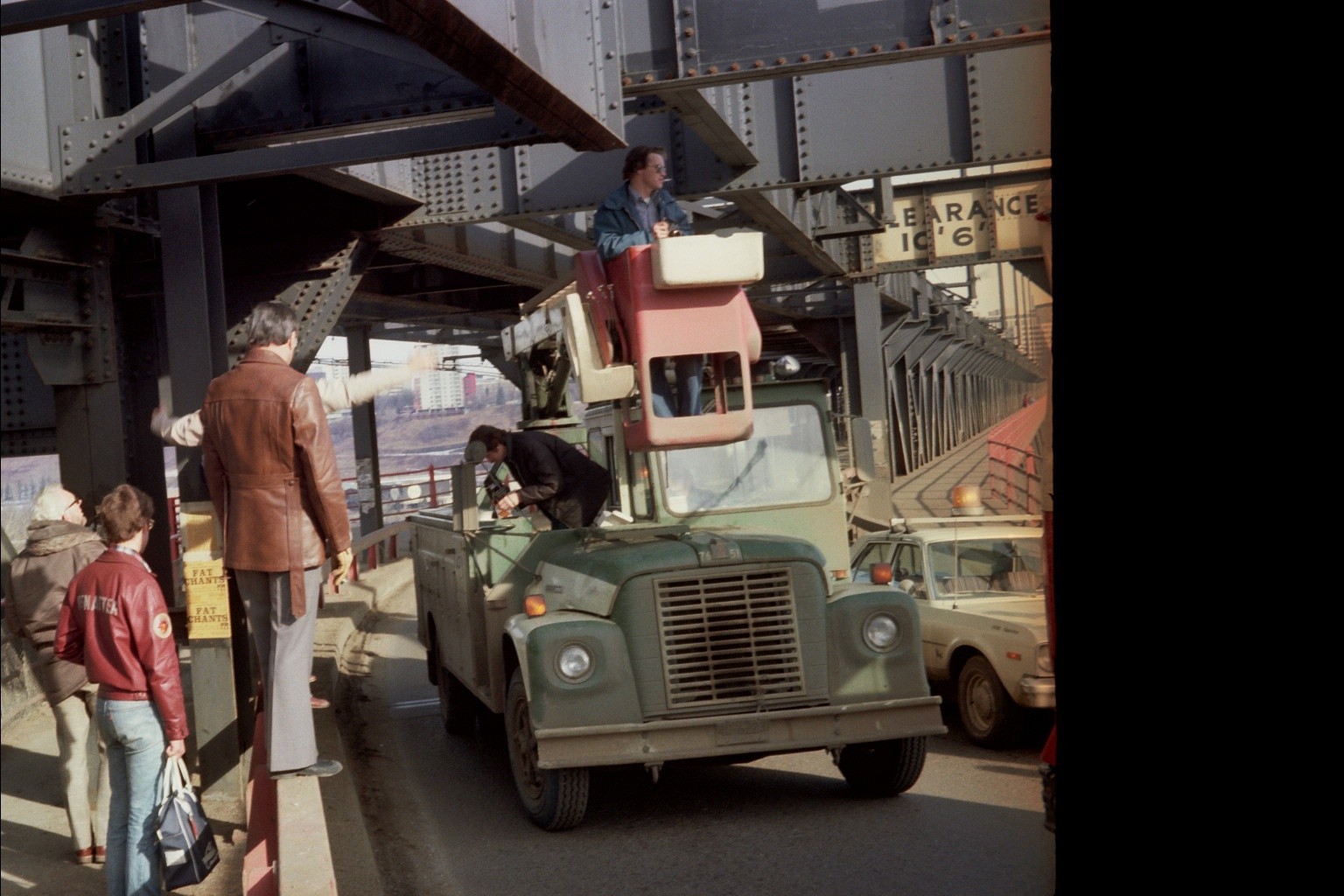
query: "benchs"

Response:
[901,570,1040,595]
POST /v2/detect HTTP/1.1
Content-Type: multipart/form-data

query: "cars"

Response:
[852,515,1056,741]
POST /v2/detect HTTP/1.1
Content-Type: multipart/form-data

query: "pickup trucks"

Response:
[406,355,949,832]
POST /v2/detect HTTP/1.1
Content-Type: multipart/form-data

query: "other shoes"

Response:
[270,759,342,780]
[312,697,330,708]
[311,675,317,683]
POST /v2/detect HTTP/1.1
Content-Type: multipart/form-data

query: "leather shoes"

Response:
[96,846,107,862]
[77,847,94,864]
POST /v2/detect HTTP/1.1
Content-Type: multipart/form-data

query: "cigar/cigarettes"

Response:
[663,178,672,182]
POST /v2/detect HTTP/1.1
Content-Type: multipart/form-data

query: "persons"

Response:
[53,485,190,896]
[469,425,613,531]
[5,485,111,863]
[151,300,442,779]
[593,144,706,418]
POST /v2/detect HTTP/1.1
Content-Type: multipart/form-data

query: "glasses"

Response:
[646,165,667,173]
[67,497,79,510]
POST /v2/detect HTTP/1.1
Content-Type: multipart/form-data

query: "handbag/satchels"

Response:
[153,754,220,892]
